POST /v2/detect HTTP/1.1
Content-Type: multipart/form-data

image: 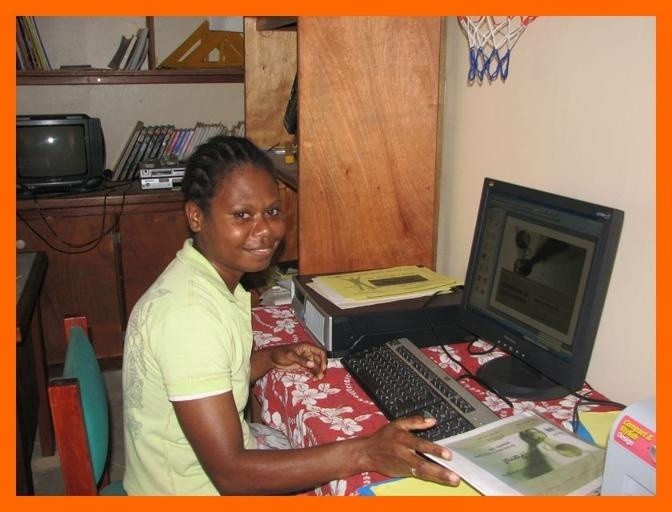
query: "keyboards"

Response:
[340,336,500,448]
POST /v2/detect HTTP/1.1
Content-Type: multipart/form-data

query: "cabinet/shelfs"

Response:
[15,62,245,375]
[242,15,445,277]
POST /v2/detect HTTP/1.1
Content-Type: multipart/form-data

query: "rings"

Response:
[411,468,416,477]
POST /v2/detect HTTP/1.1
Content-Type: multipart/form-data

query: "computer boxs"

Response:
[289,266,474,357]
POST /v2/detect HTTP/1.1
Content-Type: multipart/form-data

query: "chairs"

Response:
[46,313,130,496]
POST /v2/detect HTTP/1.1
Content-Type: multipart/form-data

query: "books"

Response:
[16,16,51,71]
[106,27,149,71]
[111,120,245,181]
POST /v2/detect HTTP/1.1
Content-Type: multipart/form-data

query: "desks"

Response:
[17,249,56,496]
[251,290,627,496]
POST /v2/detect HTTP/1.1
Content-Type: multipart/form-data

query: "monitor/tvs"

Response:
[16,115,104,196]
[456,178,625,401]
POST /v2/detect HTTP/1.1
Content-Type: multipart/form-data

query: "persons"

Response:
[121,136,460,496]
